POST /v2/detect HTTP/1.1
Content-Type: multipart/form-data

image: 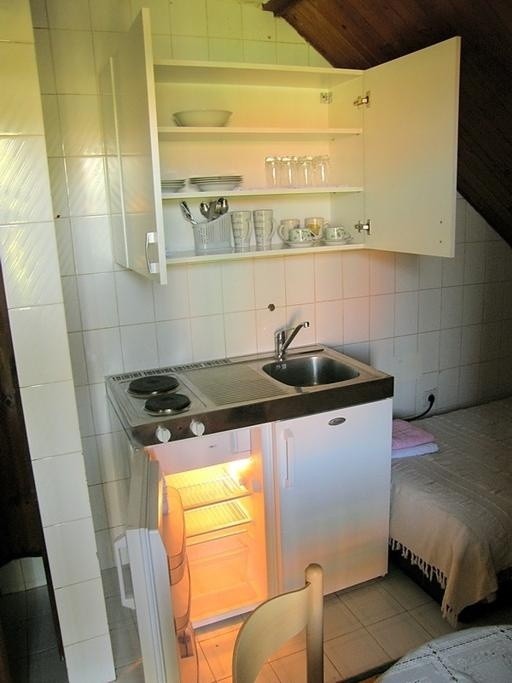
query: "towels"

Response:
[392,417,435,449]
[392,443,438,459]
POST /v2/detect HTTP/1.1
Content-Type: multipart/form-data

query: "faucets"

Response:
[281,321,310,353]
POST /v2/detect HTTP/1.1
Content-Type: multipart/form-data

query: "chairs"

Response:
[232,560,325,683]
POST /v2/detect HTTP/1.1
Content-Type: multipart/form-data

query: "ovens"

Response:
[108,423,279,682]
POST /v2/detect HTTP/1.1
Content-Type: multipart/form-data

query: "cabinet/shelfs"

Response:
[97,5,463,285]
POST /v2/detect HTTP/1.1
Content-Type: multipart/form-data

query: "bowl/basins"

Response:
[173,108,234,126]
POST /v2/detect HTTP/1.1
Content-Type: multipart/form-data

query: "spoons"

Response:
[179,197,227,223]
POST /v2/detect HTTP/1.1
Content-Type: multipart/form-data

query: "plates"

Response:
[161,178,185,192]
[287,241,314,248]
[191,174,244,189]
[325,240,350,245]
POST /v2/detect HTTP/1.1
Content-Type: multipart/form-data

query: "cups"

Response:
[252,210,275,247]
[324,226,352,242]
[288,228,318,243]
[264,153,329,187]
[230,210,253,249]
[304,217,331,241]
[279,218,300,243]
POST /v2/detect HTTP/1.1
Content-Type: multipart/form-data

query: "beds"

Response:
[389,398,510,627]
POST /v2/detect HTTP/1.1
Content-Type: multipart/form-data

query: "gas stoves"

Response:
[117,371,217,445]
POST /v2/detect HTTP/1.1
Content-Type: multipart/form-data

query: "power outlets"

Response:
[423,388,439,407]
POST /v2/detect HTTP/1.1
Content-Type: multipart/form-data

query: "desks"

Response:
[379,624,512,683]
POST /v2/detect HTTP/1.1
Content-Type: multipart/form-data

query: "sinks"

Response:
[261,354,361,387]
